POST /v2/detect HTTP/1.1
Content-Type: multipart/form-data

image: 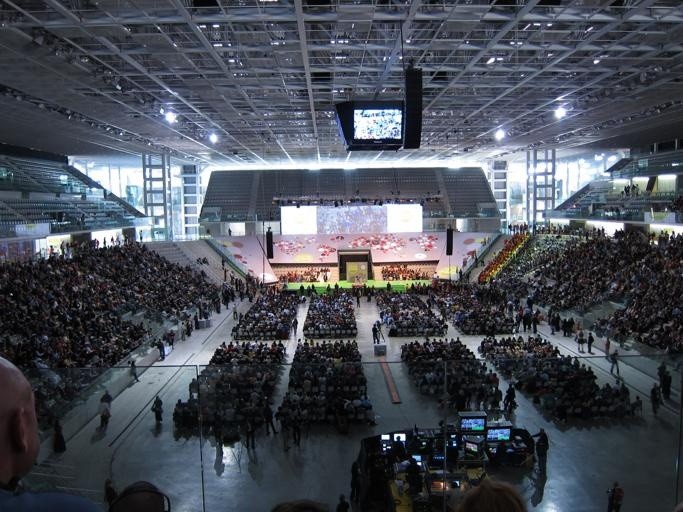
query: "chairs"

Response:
[175,293,638,427]
[562,155,681,216]
[479,231,681,354]
[199,167,498,227]
[0,242,225,392]
[0,152,137,237]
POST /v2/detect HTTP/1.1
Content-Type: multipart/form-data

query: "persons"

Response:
[0,355,105,511]
[270,499,333,511]
[108,479,171,511]
[335,493,349,512]
[605,481,624,511]
[600,181,640,217]
[453,479,530,511]
[352,108,402,141]
[80,214,85,224]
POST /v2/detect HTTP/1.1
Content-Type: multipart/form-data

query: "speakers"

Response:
[266,232,274,259]
[446,229,453,255]
[402,69,422,148]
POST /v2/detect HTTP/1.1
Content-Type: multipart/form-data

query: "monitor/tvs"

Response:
[382,413,512,466]
